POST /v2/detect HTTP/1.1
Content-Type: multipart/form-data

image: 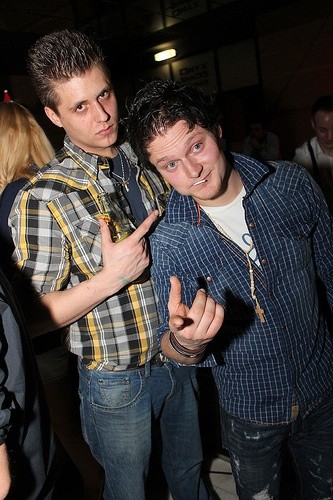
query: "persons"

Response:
[0,102,79,500]
[292,96,333,212]
[242,121,280,161]
[123,76,333,500]
[8,30,202,500]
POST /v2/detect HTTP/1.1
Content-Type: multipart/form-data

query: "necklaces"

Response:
[206,213,265,324]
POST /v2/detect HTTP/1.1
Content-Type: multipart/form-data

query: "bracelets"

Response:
[168,331,208,358]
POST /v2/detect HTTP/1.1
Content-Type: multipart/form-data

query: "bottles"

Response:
[97,191,150,284]
